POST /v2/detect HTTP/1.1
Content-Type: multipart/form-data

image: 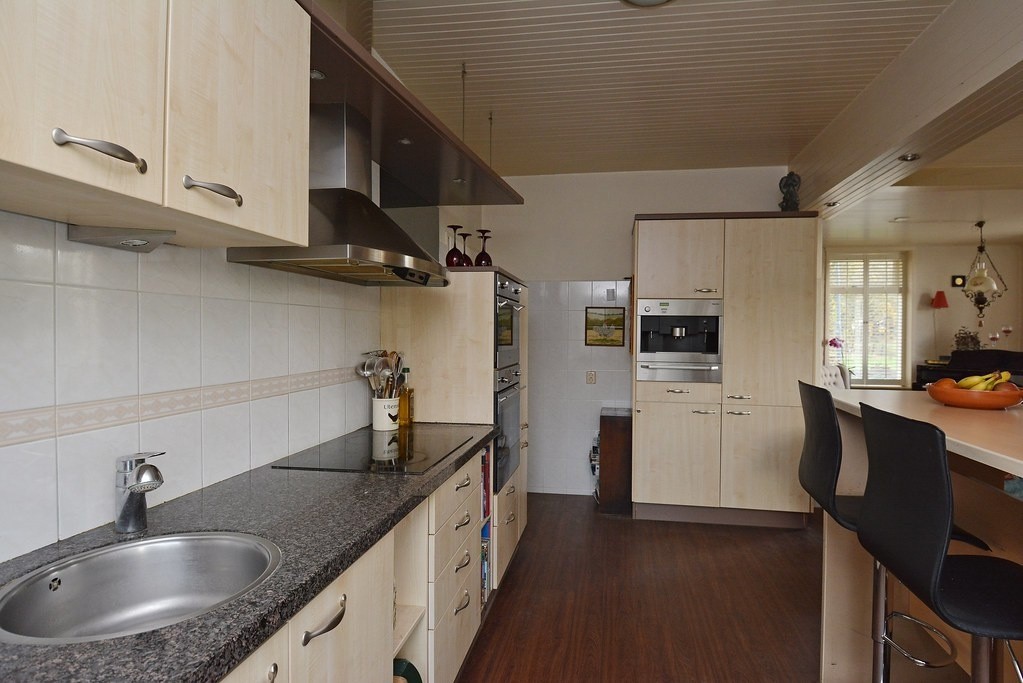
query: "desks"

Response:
[819,389,1023,683]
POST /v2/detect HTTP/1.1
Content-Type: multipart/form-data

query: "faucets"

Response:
[112,449,169,534]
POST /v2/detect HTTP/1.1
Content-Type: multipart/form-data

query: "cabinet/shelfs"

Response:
[632,217,816,527]
[589,407,632,513]
[0,0,311,253]
[219,449,482,683]
[492,284,529,590]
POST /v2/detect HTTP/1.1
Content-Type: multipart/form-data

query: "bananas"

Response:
[957,370,1011,391]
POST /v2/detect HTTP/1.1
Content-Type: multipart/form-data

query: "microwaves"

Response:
[635,299,724,383]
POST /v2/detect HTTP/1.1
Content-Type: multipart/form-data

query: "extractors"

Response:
[226,103,452,288]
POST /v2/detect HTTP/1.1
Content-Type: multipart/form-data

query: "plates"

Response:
[923,382,1023,409]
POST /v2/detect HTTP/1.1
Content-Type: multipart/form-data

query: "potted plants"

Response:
[950,325,989,351]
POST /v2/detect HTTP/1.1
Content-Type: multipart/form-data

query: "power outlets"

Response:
[585,372,596,384]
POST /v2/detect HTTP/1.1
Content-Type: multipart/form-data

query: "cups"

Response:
[372,430,400,461]
[371,397,400,432]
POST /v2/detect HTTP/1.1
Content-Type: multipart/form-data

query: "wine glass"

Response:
[457,232,474,267]
[1002,324,1013,347]
[477,235,492,266]
[445,224,464,267]
[989,330,1000,349]
[475,229,493,266]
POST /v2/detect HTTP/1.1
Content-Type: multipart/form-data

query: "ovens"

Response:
[379,265,525,493]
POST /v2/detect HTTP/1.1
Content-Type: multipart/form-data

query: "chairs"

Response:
[838,365,849,389]
[856,402,1023,683]
[822,366,846,388]
[797,379,994,683]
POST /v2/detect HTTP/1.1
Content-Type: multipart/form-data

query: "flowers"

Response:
[829,338,856,375]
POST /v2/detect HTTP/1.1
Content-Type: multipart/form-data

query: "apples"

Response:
[992,382,1019,391]
[933,377,959,389]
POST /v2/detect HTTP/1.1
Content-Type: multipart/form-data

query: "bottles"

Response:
[398,367,414,427]
[398,426,414,465]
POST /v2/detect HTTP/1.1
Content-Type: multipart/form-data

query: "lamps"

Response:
[929,291,949,361]
[961,220,1008,327]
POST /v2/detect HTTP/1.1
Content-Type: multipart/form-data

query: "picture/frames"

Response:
[585,306,625,348]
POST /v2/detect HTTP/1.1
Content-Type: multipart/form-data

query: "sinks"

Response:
[0,533,283,640]
